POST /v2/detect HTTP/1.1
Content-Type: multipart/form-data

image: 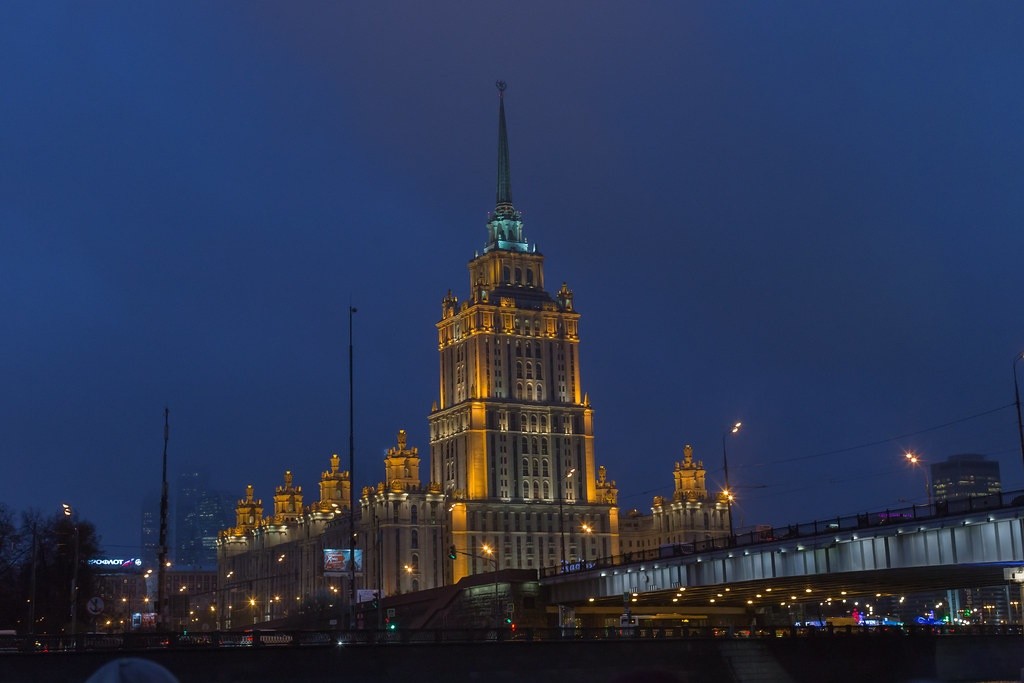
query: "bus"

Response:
[619,613,709,640]
[0,630,23,656]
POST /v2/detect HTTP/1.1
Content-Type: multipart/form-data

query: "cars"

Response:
[178,629,293,648]
[158,639,169,647]
[84,631,123,650]
[710,618,1024,639]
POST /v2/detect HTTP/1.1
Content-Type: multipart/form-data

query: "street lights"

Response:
[481,543,502,643]
[720,419,743,549]
[127,558,142,631]
[227,571,233,630]
[904,448,937,517]
[63,504,78,648]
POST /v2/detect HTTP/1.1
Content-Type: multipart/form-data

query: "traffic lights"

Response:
[506,618,512,624]
[511,624,515,631]
[449,553,456,559]
[390,623,396,631]
[184,628,187,636]
